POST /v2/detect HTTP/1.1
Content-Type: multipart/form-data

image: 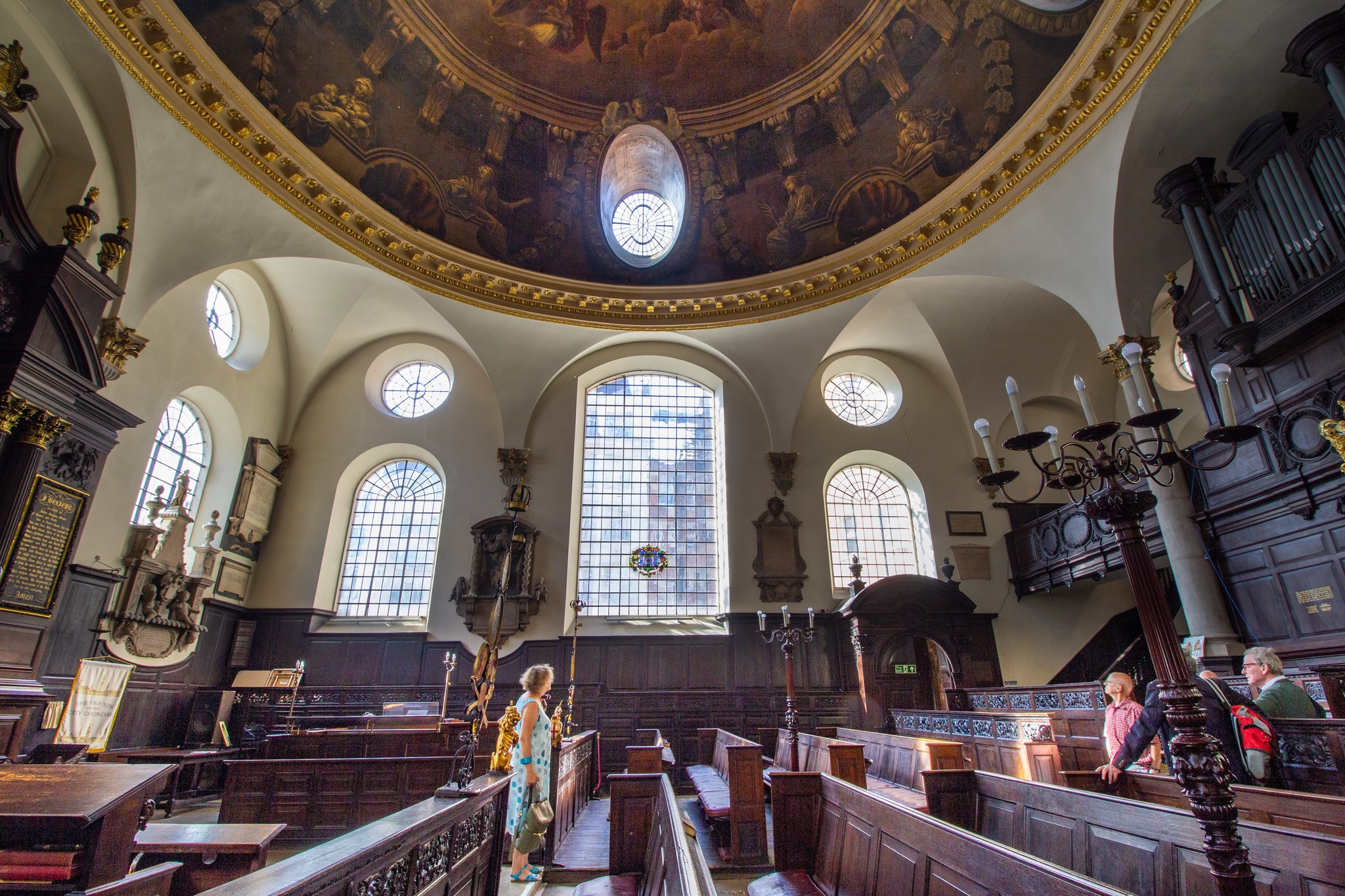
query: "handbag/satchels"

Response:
[514,780,554,854]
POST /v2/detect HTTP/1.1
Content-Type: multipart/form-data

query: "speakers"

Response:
[184,691,237,745]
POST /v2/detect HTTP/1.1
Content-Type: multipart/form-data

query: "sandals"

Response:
[524,862,544,874]
[510,868,542,883]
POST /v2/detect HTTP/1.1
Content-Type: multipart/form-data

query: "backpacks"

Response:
[1203,676,1280,778]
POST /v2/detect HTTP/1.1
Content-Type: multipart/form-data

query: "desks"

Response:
[117,747,258,818]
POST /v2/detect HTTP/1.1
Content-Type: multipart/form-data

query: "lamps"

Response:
[285,660,305,735]
[442,652,458,723]
[757,605,818,771]
[973,342,1260,896]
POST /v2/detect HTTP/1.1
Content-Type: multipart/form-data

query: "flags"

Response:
[53,659,133,753]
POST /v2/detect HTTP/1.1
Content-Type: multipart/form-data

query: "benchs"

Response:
[606,681,1345,896]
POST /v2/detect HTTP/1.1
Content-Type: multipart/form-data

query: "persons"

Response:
[166,591,203,633]
[505,663,554,882]
[1103,672,1162,777]
[113,621,137,645]
[482,534,497,551]
[174,631,199,653]
[486,552,518,571]
[448,576,465,602]
[534,577,549,602]
[1199,671,1218,679]
[1095,644,1267,784]
[170,469,192,506]
[1241,648,1316,720]
[142,584,162,624]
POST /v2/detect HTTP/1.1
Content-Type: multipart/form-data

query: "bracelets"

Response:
[520,756,532,764]
[1149,766,1161,774]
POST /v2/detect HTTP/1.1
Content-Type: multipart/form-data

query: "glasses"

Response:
[1241,663,1255,670]
[1104,679,1120,685]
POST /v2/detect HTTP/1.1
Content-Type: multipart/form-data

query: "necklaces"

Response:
[533,695,541,702]
